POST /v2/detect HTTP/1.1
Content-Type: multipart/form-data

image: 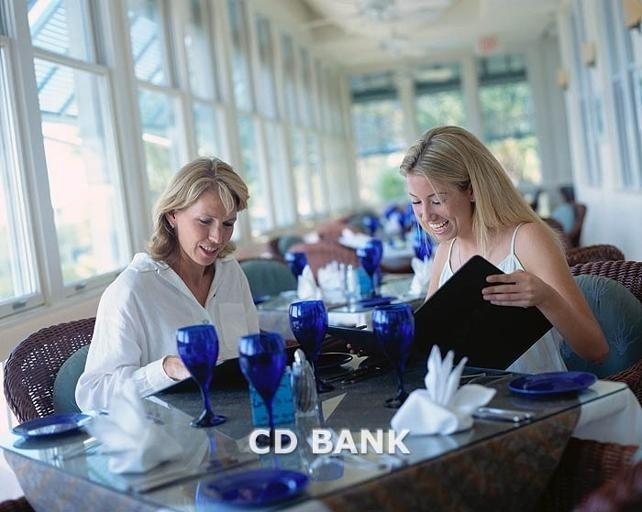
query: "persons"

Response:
[397,125,612,377]
[549,185,577,236]
[75,157,262,415]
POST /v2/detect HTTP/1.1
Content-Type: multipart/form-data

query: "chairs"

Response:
[0,199,642,512]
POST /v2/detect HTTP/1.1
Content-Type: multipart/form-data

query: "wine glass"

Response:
[358,240,391,310]
[177,298,415,450]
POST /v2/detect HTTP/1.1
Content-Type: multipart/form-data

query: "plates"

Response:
[11,413,93,441]
[193,468,312,507]
[508,370,597,399]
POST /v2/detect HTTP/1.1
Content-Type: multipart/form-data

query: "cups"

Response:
[362,214,379,238]
[415,231,433,260]
[287,253,308,277]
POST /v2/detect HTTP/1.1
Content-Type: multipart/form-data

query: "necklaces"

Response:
[455,241,462,268]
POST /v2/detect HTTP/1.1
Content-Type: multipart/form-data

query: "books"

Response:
[327,254,553,371]
[140,324,367,397]
